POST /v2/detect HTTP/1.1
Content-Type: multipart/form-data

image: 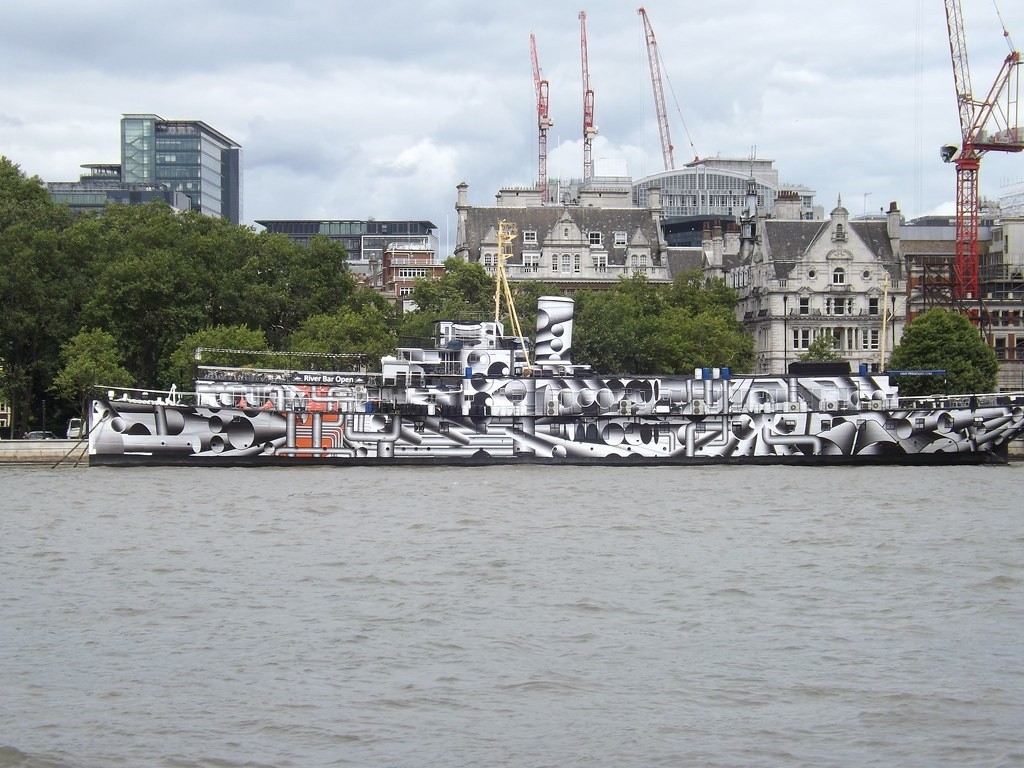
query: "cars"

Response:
[26,430,58,441]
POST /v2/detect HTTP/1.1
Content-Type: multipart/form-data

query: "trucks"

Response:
[67,418,90,440]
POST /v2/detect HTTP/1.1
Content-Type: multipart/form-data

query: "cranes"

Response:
[528,31,555,199]
[576,9,600,186]
[937,0,1023,303]
[634,6,697,170]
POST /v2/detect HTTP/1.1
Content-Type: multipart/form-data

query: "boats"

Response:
[90,295,1024,466]
